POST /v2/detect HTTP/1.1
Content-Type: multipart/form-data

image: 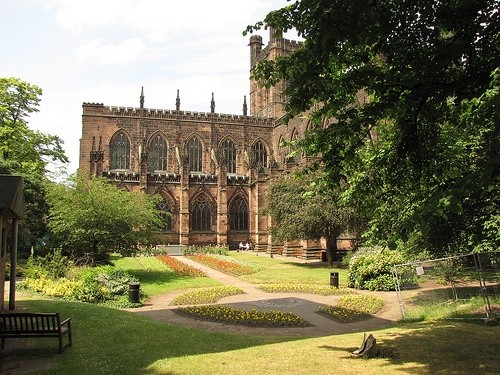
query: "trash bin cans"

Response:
[330,272,339,288]
[129,283,140,303]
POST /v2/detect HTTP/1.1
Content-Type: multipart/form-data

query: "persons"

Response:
[245,242,250,251]
[239,242,246,250]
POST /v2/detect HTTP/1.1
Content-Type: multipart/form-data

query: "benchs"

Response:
[0,311,73,354]
[252,243,351,262]
